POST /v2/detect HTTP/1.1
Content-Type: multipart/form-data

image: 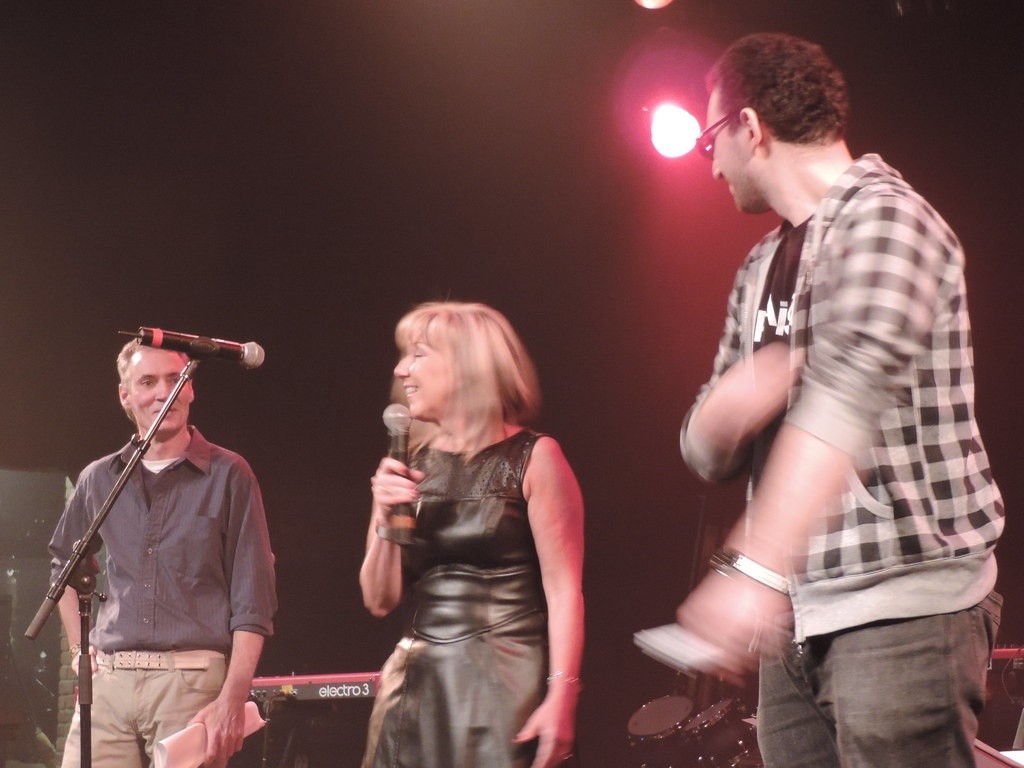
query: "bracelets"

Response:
[67,640,81,665]
[544,670,582,689]
[710,546,798,599]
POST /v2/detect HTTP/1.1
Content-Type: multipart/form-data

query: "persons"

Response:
[671,31,1008,768]
[358,300,589,766]
[45,338,278,766]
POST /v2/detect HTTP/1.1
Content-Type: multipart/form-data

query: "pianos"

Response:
[247,671,382,703]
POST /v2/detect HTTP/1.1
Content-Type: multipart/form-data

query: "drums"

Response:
[625,694,696,768]
[683,693,757,768]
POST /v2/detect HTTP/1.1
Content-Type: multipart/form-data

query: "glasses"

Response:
[695,106,742,160]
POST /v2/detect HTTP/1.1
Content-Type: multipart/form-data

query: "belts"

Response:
[96,649,226,669]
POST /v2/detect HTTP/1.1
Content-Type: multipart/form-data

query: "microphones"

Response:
[119,325,265,370]
[382,404,417,542]
